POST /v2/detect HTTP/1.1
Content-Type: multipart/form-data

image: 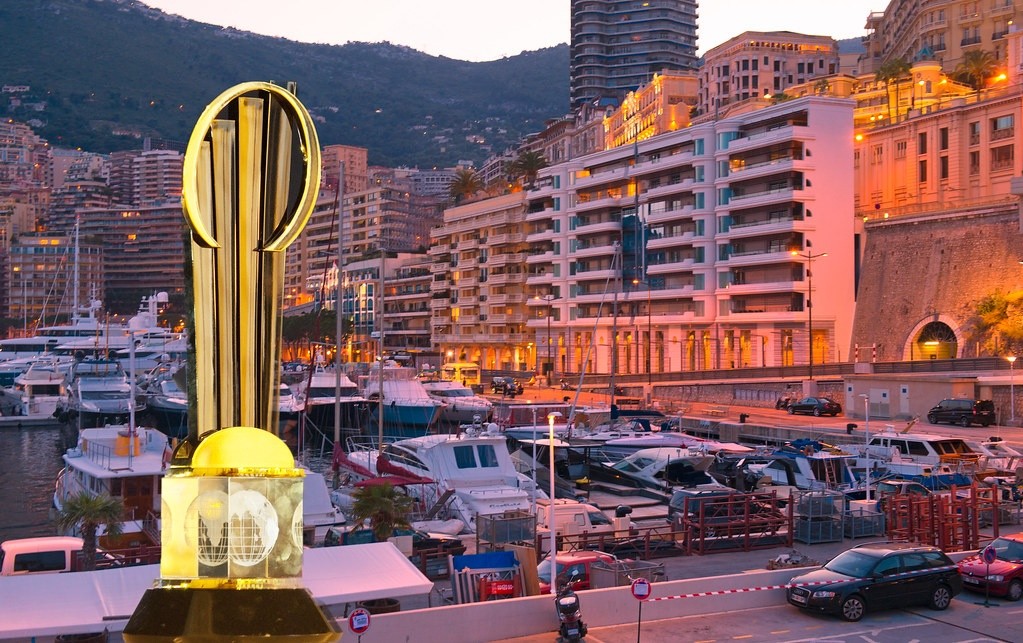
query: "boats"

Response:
[0,214,494,438]
[298,465,347,545]
[346,240,1023,530]
[53,331,175,547]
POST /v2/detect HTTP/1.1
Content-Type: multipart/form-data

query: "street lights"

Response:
[858,394,871,501]
[549,411,565,595]
[1008,356,1016,421]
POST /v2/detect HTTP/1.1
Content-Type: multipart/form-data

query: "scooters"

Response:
[775,394,790,409]
[539,575,589,643]
[560,378,571,391]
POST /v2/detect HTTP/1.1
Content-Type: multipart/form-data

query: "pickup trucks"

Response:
[538,551,635,595]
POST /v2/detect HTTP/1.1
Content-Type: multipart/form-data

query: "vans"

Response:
[926,398,996,428]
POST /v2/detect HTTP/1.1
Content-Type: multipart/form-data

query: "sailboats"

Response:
[299,156,466,537]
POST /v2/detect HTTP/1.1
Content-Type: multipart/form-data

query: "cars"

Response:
[874,479,936,504]
[786,397,842,417]
[954,532,1023,599]
[0,536,135,577]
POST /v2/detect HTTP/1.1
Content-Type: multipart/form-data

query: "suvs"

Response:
[784,537,964,620]
[491,376,524,395]
[323,522,467,568]
[664,482,784,535]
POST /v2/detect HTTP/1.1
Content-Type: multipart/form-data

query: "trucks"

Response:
[529,499,640,549]
